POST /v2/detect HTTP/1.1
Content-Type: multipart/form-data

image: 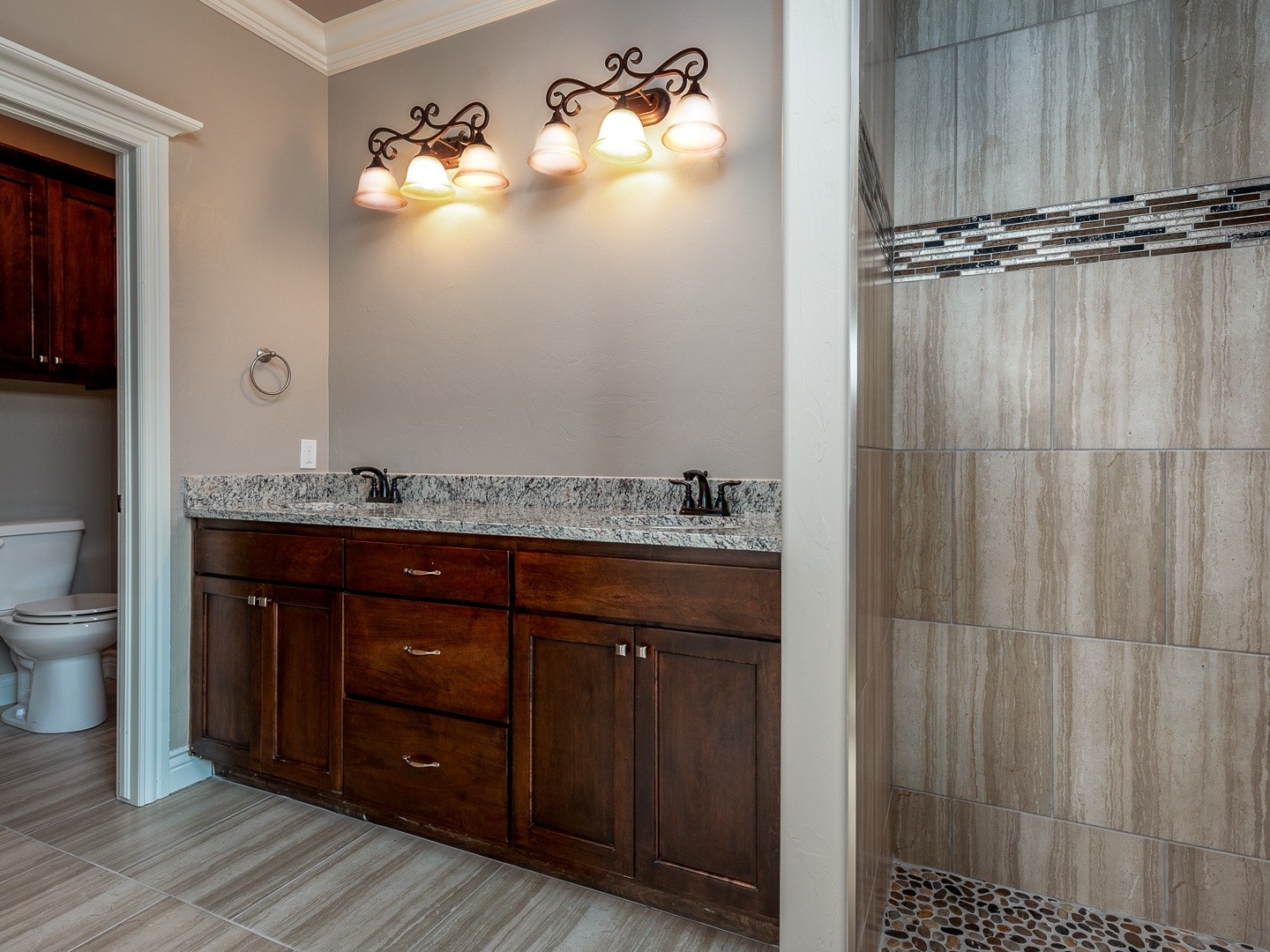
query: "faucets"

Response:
[667,469,742,516]
[351,464,408,503]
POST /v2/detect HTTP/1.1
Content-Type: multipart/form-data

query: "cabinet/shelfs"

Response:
[188,519,783,948]
[0,141,120,391]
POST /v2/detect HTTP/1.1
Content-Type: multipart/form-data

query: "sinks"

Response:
[284,502,397,511]
[600,515,745,528]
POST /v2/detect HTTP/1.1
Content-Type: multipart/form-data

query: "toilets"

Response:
[0,516,118,733]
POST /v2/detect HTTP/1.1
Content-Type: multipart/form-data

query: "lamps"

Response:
[526,48,732,176]
[352,104,507,209]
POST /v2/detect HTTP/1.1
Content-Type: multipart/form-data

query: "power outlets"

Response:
[300,440,318,469]
